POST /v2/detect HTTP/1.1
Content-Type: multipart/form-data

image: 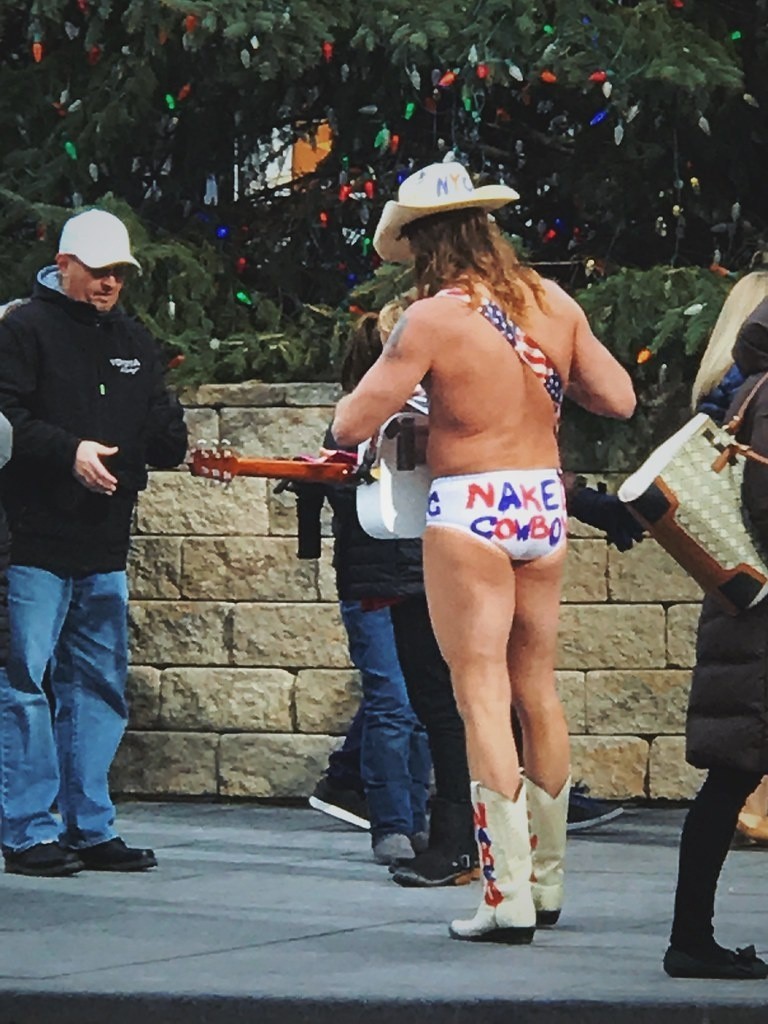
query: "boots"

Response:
[450,780,537,944]
[394,795,480,885]
[523,764,571,924]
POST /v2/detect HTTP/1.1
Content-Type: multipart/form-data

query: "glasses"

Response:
[69,254,125,279]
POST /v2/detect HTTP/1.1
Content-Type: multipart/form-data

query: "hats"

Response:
[373,161,520,262]
[60,208,141,270]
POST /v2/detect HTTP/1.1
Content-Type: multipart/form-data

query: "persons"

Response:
[309,162,637,947]
[664,268,768,979]
[1,211,190,877]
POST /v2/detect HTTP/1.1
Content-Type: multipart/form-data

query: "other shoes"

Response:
[567,782,626,829]
[309,775,370,828]
[662,944,768,979]
[374,831,430,864]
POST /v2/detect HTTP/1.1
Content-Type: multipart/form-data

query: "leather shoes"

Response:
[2,843,83,876]
[66,835,157,872]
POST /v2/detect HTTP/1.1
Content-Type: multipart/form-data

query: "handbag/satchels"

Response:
[618,365,768,609]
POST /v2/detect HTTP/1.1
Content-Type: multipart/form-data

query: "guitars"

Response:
[186,412,430,540]
